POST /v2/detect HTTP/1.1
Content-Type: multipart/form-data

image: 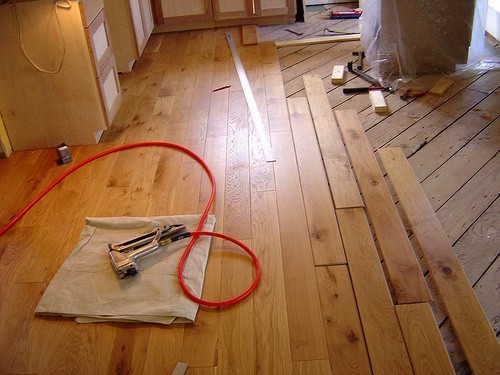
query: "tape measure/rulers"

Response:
[224,29,276,163]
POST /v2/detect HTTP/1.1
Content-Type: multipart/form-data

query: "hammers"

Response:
[351,51,366,69]
[343,80,394,94]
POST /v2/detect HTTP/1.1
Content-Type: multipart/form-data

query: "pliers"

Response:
[399,87,426,101]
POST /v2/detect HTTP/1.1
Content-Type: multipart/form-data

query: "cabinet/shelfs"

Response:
[150,0,297,33]
[0,0,121,144]
[102,0,154,73]
